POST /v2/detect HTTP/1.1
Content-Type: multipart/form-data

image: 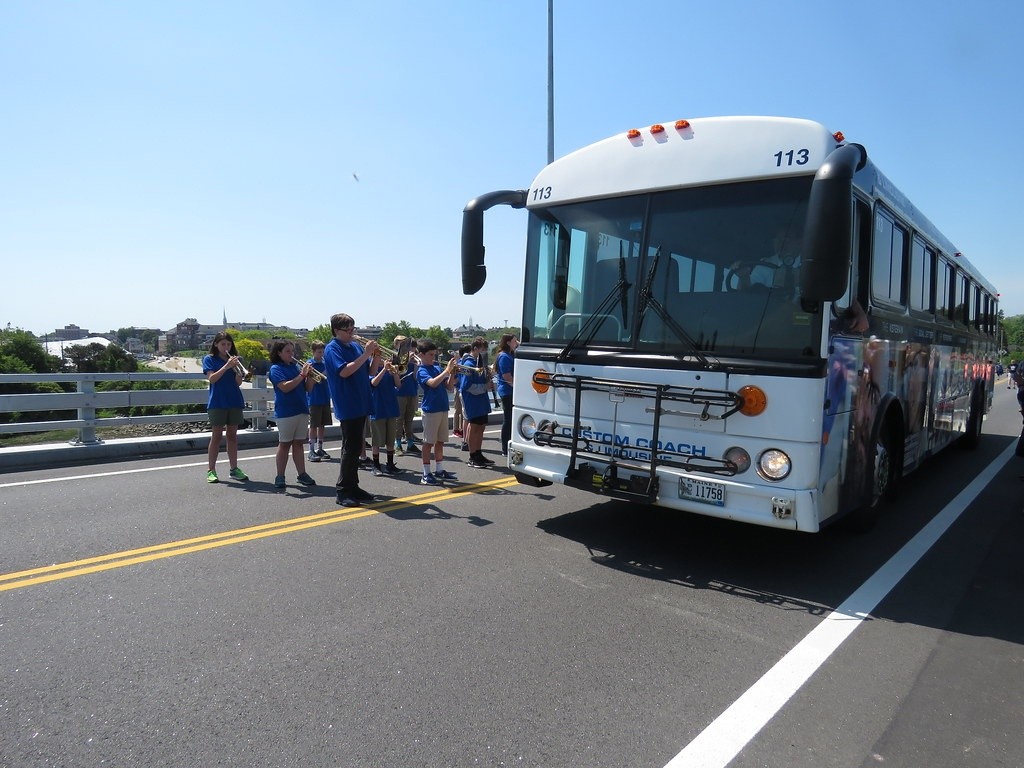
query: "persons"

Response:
[495,332,520,457]
[203,332,248,483]
[268,340,316,488]
[358,335,495,476]
[324,314,381,506]
[732,219,869,332]
[305,339,332,461]
[846,335,993,469]
[416,342,457,486]
[996,359,1024,478]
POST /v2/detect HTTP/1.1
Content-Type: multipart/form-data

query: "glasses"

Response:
[338,327,357,334]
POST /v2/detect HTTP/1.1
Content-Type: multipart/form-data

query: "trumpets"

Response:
[351,334,409,365]
[433,359,484,377]
[291,357,328,383]
[225,349,252,380]
[379,358,408,374]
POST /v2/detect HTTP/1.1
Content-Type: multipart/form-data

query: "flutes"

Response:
[486,373,500,409]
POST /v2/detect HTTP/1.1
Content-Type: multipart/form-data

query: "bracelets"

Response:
[445,368,450,375]
[299,372,305,378]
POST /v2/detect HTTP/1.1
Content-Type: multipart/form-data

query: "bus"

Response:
[459,113,1007,537]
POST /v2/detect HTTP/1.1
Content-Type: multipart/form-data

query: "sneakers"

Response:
[207,470,218,482]
[352,486,373,503]
[502,453,508,457]
[229,468,248,480]
[317,450,330,459]
[335,494,360,506]
[371,465,383,475]
[385,462,404,473]
[405,435,423,443]
[421,472,443,486]
[474,462,490,468]
[453,430,464,438]
[308,452,321,462]
[274,475,286,492]
[461,441,469,452]
[467,458,495,466]
[395,445,404,456]
[406,443,421,455]
[358,456,374,470]
[297,472,315,485]
[365,441,372,449]
[435,469,457,481]
